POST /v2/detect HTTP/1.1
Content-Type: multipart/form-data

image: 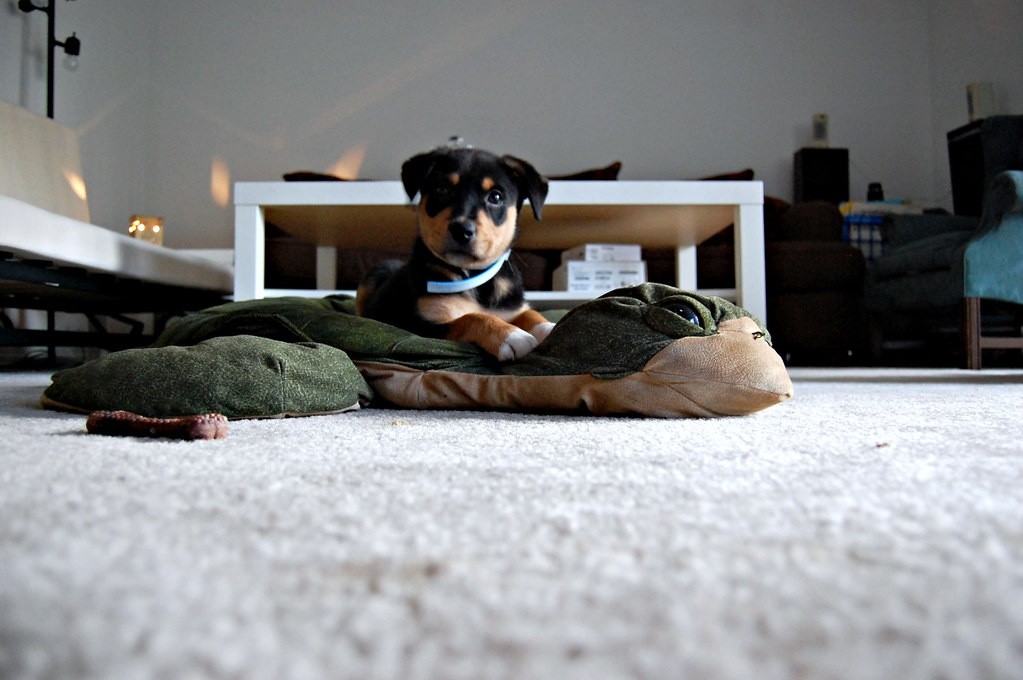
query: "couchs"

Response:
[265,157,865,371]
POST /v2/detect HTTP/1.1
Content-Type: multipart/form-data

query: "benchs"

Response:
[1,98,234,370]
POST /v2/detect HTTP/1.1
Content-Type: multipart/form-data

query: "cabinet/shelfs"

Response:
[947,115,1023,213]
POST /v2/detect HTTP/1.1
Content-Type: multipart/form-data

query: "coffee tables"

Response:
[233,177,766,363]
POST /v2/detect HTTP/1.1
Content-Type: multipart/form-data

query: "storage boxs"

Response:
[553,239,647,293]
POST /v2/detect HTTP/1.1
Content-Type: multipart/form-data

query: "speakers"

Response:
[946,112,1023,216]
[792,148,849,207]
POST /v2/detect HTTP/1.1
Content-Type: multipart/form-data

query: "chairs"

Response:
[865,169,1023,369]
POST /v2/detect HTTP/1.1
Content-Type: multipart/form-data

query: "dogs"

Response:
[353,144,564,363]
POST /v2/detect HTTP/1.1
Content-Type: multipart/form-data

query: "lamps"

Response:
[20,0,80,118]
